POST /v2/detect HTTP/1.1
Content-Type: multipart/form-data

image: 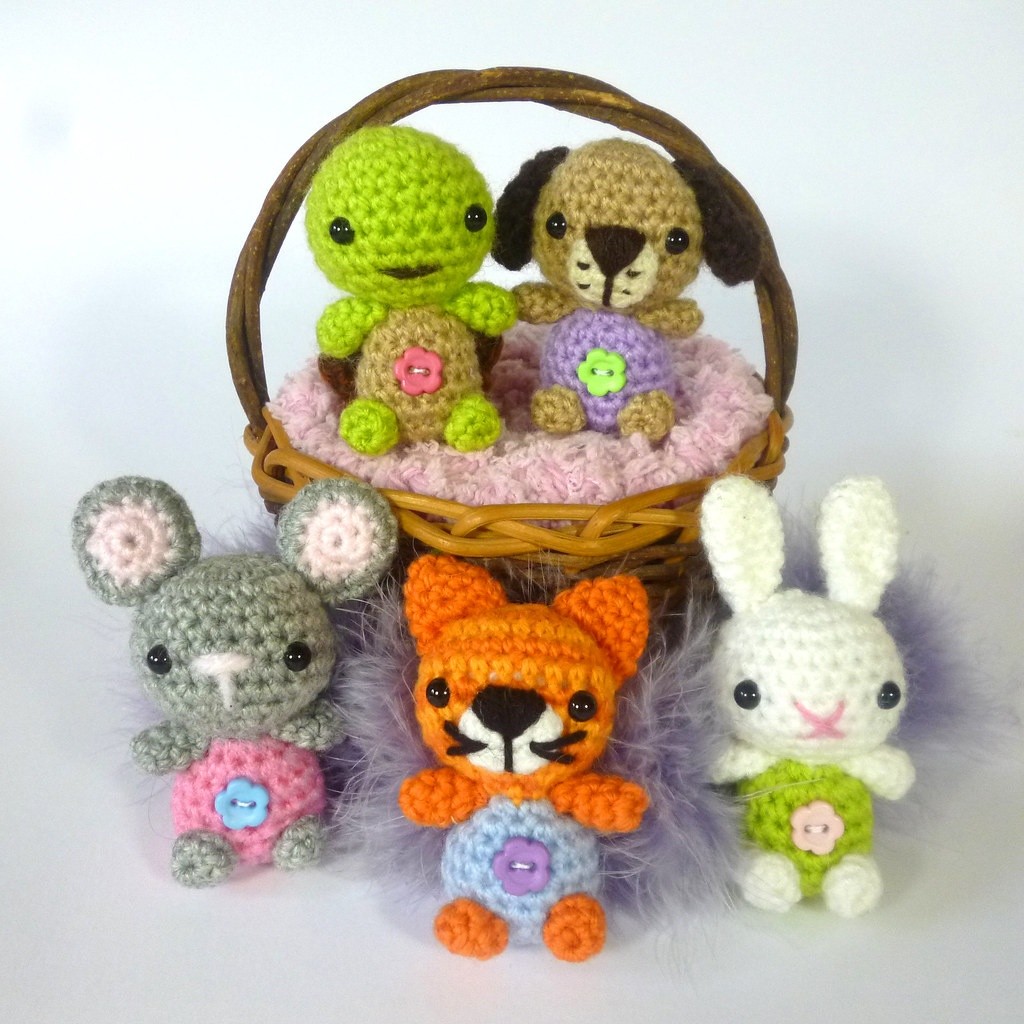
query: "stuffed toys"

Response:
[302,123,520,458]
[490,137,760,446]
[698,474,919,918]
[397,555,652,964]
[69,472,398,889]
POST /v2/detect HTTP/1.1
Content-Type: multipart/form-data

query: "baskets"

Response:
[225,70,799,620]
